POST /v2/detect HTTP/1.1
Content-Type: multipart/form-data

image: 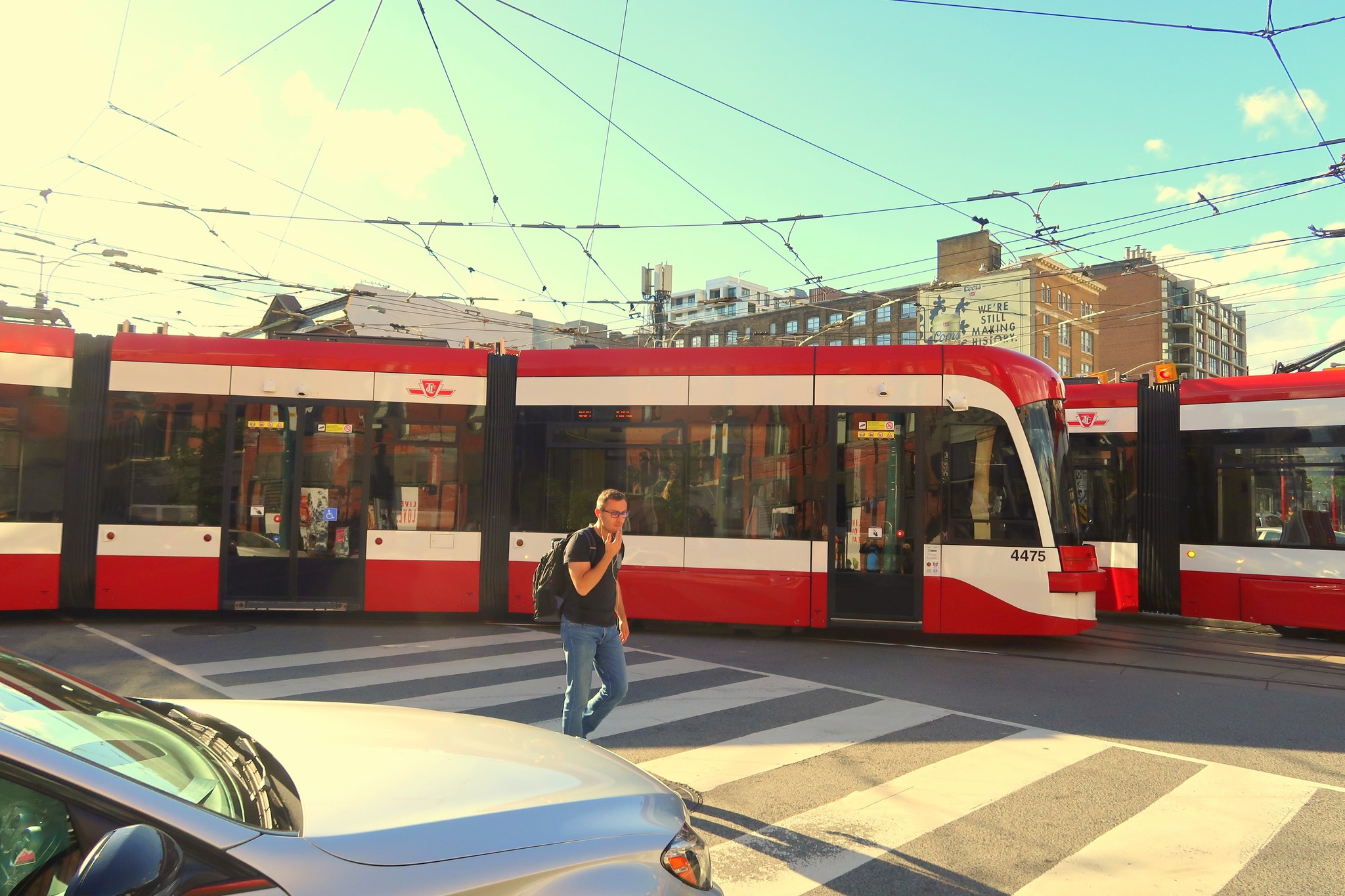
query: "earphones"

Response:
[599,513,601,520]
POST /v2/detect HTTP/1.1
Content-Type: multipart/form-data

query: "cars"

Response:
[0,639,721,896]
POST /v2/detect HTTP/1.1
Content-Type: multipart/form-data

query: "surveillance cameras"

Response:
[878,383,889,396]
[297,384,306,396]
[944,397,968,412]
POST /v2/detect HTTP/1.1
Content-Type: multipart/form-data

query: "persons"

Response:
[1087,468,1129,542]
[558,489,629,739]
[1287,507,1294,521]
[859,537,882,573]
[629,457,716,538]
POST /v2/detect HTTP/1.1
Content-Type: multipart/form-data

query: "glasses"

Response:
[599,509,631,518]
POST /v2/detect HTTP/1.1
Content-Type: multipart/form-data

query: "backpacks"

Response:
[532,530,596,623]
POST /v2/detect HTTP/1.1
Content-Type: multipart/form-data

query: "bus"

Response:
[0,326,1104,639]
[1061,339,1345,637]
